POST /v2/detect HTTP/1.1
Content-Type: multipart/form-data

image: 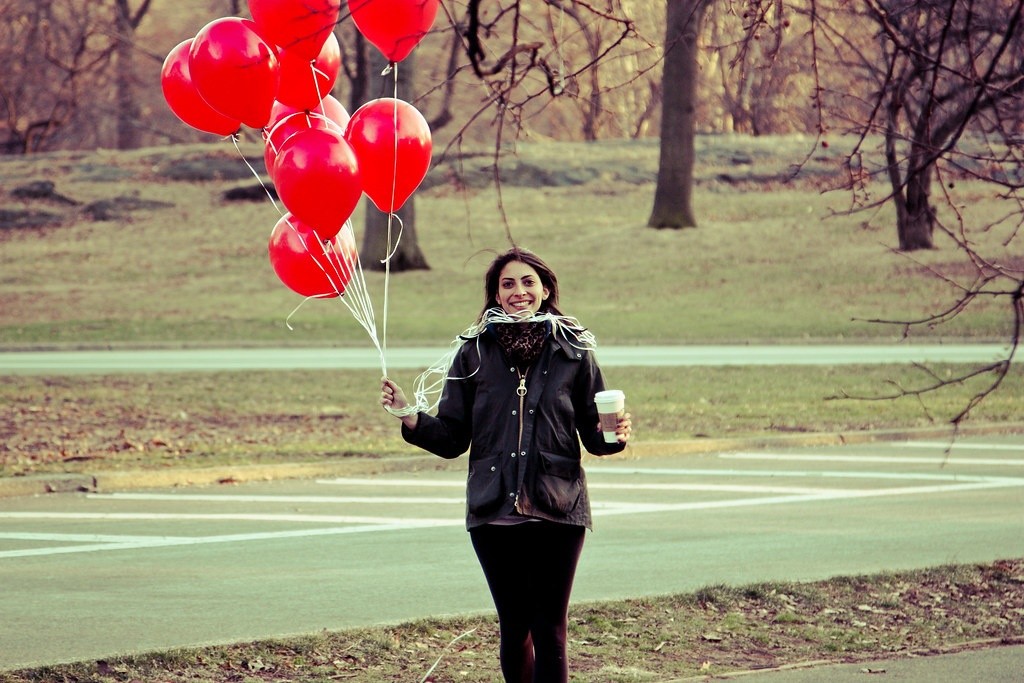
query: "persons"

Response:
[380,246,633,683]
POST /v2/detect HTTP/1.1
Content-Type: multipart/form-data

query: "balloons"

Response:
[161,0,438,298]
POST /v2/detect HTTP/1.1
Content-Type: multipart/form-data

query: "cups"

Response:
[594,390,625,443]
